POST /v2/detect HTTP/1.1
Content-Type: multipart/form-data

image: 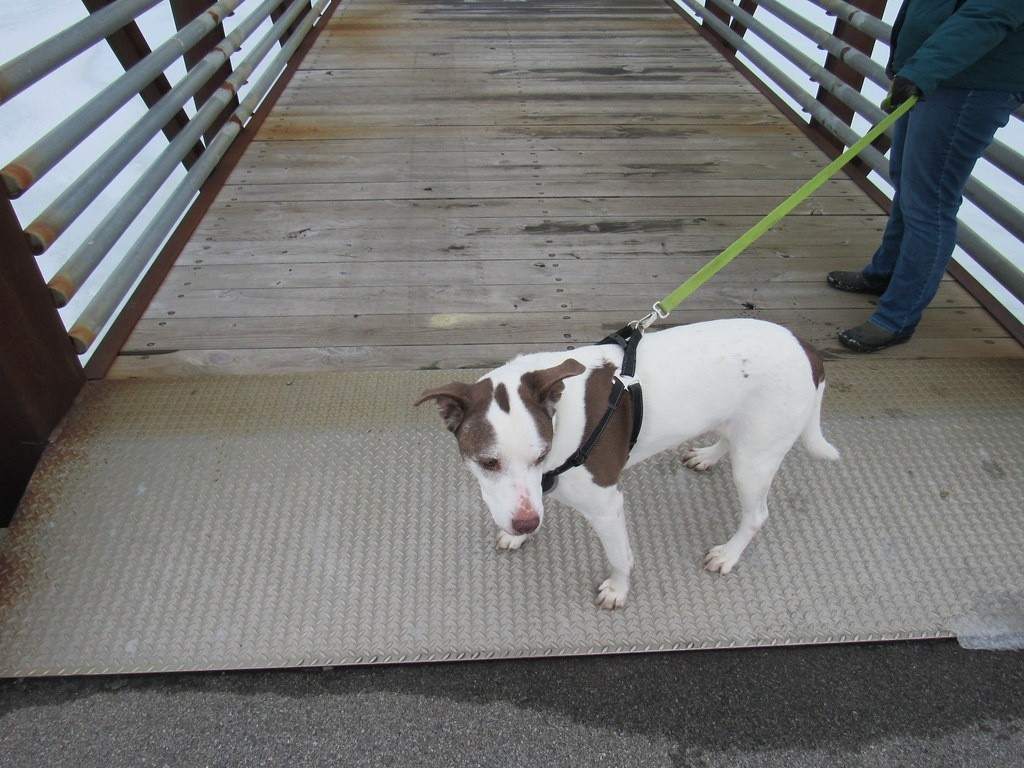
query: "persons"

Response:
[828,1,1024,352]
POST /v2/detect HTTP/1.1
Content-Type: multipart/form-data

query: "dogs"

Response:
[411,317,842,611]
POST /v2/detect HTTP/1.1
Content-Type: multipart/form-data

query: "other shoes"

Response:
[829,269,879,294]
[839,319,913,352]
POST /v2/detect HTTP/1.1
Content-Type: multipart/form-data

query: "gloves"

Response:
[880,76,922,116]
